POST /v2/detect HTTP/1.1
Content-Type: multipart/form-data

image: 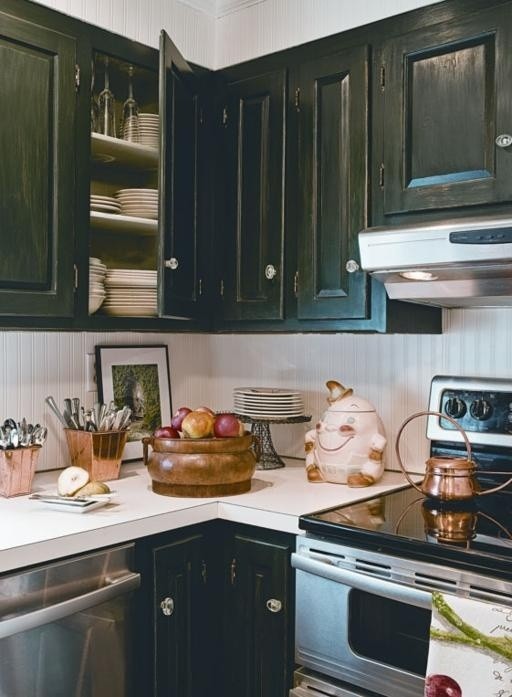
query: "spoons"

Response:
[0,418,48,450]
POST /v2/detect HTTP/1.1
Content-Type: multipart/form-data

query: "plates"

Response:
[27,488,119,513]
[88,187,159,220]
[89,257,158,320]
[232,386,305,420]
[119,112,160,149]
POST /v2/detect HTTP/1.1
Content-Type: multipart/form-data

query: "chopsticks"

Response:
[29,493,113,503]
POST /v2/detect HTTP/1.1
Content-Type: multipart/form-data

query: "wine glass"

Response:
[96,55,117,137]
[117,62,142,144]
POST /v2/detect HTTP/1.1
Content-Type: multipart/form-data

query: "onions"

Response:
[426,675,461,696]
[153,406,246,439]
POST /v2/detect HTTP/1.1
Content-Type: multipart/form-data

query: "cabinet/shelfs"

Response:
[213,23,438,333]
[381,0,511,224]
[141,520,295,696]
[1,1,212,332]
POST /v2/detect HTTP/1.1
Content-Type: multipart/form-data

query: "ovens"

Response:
[288,531,511,696]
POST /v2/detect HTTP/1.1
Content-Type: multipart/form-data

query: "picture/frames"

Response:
[98,346,173,462]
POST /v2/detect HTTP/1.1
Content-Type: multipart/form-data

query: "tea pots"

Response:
[395,411,512,501]
[394,496,512,548]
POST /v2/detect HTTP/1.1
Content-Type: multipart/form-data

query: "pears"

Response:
[58,466,109,496]
